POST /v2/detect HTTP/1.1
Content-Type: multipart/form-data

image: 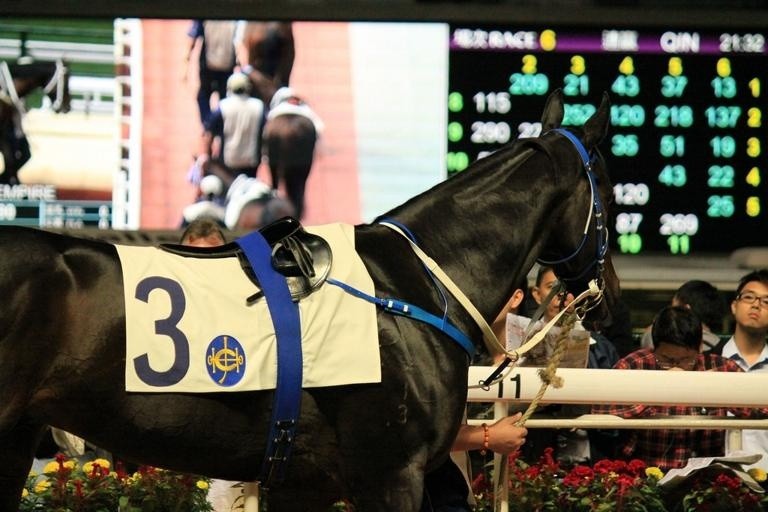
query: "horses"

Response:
[241,64,316,221]
[0,57,72,185]
[190,153,300,232]
[240,20,294,88]
[0,89,625,512]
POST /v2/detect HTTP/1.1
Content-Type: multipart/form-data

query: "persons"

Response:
[485,263,768,492]
[450,409,530,457]
[178,18,237,129]
[182,175,226,227]
[189,73,267,191]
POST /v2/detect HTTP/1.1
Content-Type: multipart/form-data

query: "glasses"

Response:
[655,357,697,370]
[736,290,768,308]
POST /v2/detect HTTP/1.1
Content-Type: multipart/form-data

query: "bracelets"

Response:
[477,421,491,458]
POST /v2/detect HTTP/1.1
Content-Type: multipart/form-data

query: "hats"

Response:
[227,73,250,94]
[199,175,222,198]
